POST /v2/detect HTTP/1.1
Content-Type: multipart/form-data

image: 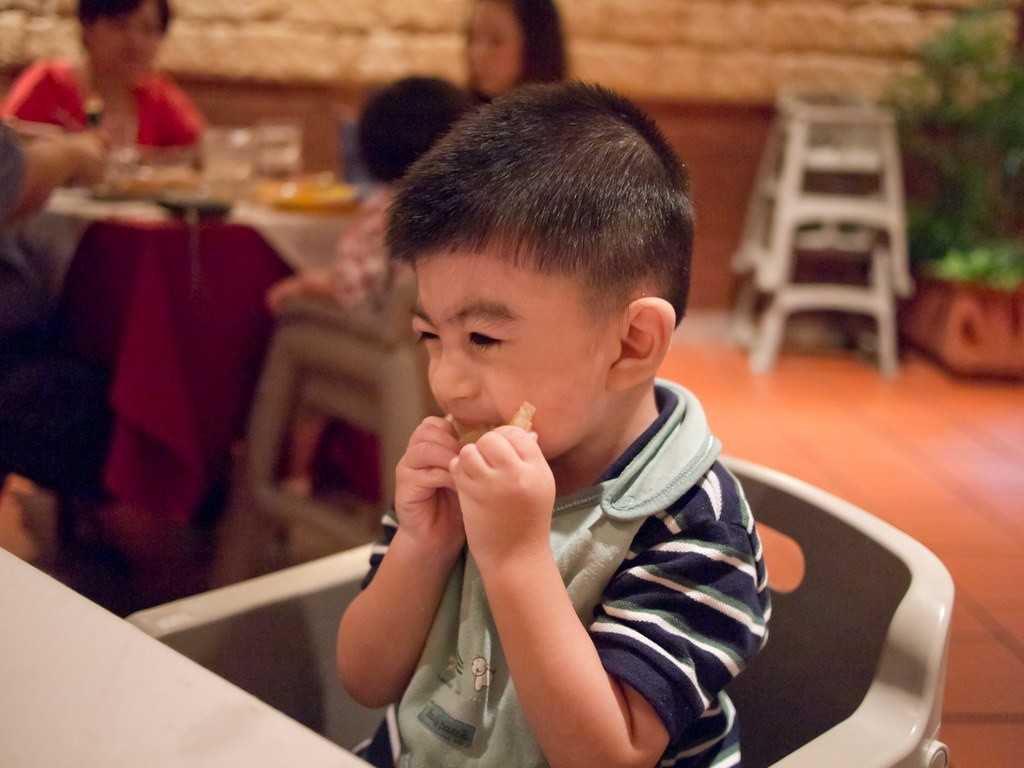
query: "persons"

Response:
[263,78,472,502]
[333,0,569,197]
[337,78,773,768]
[0,0,214,168]
[0,113,112,587]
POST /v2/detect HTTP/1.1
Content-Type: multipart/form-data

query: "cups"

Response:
[257,123,302,178]
[202,128,255,183]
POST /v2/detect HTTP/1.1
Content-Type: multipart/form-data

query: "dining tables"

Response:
[11,183,372,563]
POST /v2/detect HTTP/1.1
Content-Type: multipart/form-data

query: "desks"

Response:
[1,548,374,768]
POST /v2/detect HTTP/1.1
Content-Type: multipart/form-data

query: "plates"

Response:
[158,190,230,218]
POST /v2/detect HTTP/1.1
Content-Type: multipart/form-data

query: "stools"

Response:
[730,85,914,382]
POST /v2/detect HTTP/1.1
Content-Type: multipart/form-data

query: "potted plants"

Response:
[898,238,1020,382]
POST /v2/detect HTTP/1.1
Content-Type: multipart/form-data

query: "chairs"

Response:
[120,454,957,768]
[203,257,446,592]
